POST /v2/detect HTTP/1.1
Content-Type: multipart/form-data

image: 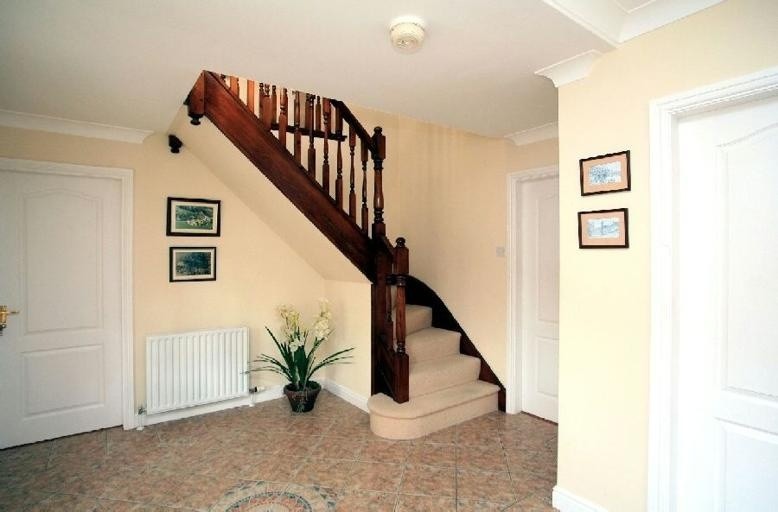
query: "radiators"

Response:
[146,326,249,415]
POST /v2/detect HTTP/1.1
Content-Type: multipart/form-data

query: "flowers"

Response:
[241,298,354,389]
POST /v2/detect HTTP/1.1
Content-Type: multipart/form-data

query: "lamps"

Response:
[389,22,425,54]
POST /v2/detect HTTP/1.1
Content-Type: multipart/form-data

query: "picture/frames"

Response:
[166,196,221,236]
[169,247,217,282]
[577,206,629,250]
[578,150,630,196]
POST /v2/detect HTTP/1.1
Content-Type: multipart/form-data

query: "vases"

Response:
[282,381,321,413]
